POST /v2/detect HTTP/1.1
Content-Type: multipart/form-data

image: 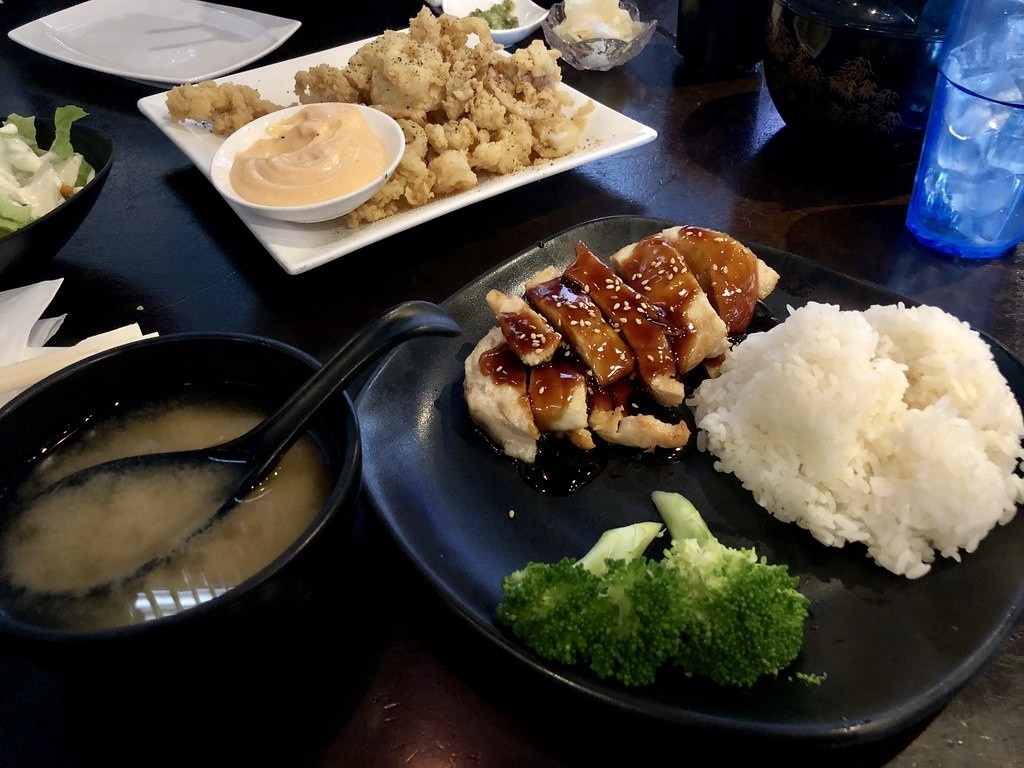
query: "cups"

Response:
[677,1,769,71]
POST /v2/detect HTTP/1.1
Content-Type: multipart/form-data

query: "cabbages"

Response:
[0,105,96,239]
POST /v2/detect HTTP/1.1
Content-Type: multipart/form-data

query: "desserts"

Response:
[229,102,389,208]
[552,0,644,43]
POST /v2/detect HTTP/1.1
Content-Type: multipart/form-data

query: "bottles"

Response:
[903,0,1023,259]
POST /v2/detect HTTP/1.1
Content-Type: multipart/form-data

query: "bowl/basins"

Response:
[441,0,549,48]
[769,1,947,150]
[209,105,406,222]
[541,0,657,71]
[0,94,115,285]
[1,330,363,705]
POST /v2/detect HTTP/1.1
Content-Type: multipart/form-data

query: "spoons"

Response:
[0,297,462,603]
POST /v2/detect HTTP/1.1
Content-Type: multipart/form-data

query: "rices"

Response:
[686,299,1024,580]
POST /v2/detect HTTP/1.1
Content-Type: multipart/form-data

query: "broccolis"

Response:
[495,491,807,689]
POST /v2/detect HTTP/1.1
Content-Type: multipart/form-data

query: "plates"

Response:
[137,28,657,277]
[7,0,302,90]
[354,214,1024,736]
